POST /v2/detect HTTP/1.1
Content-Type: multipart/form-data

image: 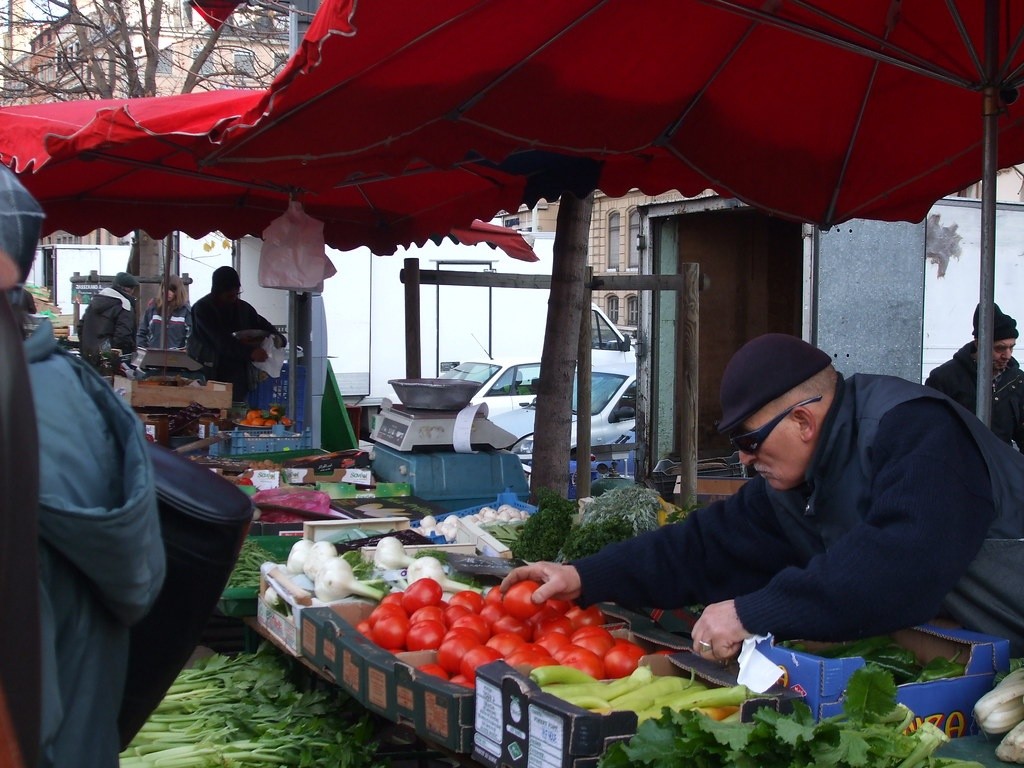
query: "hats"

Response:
[971,302,1019,342]
[1,163,47,290]
[717,333,833,433]
[115,272,140,288]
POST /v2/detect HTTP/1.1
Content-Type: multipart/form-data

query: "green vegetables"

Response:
[116,640,418,768]
[513,486,701,564]
[597,657,1024,768]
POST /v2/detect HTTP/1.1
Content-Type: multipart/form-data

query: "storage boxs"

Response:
[113,375,232,409]
[738,623,1010,740]
[299,602,654,704]
[409,487,538,544]
[247,520,304,537]
[245,363,306,433]
[209,423,312,456]
[472,651,803,768]
[361,623,693,754]
[257,571,303,657]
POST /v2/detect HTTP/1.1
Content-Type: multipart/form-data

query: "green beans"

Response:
[224,538,285,589]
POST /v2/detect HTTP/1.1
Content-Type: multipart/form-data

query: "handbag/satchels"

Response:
[251,335,303,379]
[120,442,254,750]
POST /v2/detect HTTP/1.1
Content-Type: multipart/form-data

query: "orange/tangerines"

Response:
[239,407,290,427]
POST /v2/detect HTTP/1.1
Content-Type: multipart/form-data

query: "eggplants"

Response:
[334,527,433,553]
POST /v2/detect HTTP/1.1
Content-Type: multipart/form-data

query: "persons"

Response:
[187,266,287,403]
[925,303,1024,455]
[76,272,139,375]
[0,170,166,768]
[141,274,193,351]
[499,333,1024,666]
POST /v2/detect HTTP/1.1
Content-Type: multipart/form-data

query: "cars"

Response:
[489,361,636,476]
[435,357,541,418]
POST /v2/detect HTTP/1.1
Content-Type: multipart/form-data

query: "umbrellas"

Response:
[207,0,1024,433]
[0,86,539,425]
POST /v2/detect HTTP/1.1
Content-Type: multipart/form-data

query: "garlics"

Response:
[286,535,447,602]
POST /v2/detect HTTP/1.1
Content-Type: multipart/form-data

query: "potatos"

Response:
[224,459,281,487]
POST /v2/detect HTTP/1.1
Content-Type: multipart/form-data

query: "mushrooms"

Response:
[413,504,532,542]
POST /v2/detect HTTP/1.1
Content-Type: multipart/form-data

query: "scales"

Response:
[367,378,521,450]
[131,347,204,371]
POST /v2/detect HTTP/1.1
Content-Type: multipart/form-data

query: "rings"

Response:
[698,640,712,652]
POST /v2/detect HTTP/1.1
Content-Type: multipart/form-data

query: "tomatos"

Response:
[355,580,676,689]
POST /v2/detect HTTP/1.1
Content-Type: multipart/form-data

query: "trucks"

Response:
[228,227,637,408]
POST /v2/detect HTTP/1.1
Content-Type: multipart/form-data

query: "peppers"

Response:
[527,665,785,727]
[782,634,968,685]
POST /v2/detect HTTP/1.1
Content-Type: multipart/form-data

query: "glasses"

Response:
[729,396,822,455]
[225,285,243,298]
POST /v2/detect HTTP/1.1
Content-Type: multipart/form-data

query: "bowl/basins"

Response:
[388,379,484,410]
[169,435,199,451]
[232,418,295,430]
